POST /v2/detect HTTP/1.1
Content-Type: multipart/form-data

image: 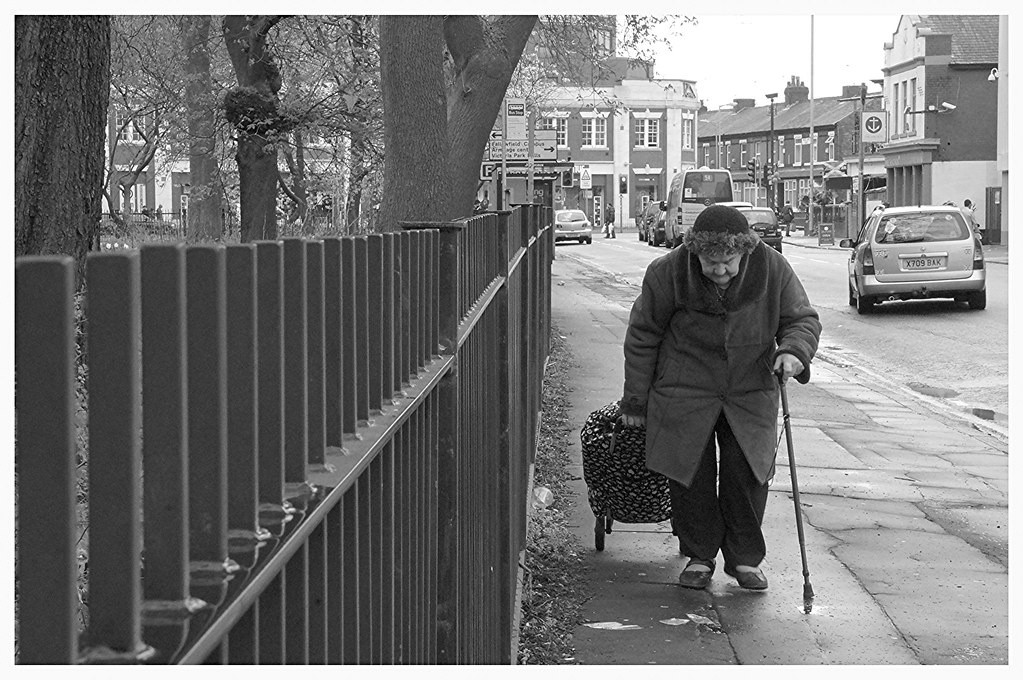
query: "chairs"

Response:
[894,222,912,234]
[926,219,952,233]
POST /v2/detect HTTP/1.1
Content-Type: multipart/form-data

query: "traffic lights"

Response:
[747,158,756,184]
[764,165,773,187]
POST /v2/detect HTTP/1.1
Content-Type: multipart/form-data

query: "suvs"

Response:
[840,200,990,315]
[637,200,668,242]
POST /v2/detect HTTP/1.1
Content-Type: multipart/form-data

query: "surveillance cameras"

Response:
[942,102,957,110]
[904,106,911,114]
[988,74,996,83]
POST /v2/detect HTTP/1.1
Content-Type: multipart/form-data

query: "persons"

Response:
[781,200,794,237]
[616,205,824,592]
[961,200,979,229]
[604,203,616,239]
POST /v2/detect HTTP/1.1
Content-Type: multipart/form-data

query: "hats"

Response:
[683,205,760,255]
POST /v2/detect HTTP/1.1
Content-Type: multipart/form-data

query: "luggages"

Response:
[581,399,678,551]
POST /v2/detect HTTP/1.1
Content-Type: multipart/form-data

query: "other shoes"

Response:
[724,564,768,590]
[679,558,716,588]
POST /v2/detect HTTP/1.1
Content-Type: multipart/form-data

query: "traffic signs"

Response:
[489,130,558,163]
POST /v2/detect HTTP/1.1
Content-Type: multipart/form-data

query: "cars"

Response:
[646,210,667,247]
[554,209,593,245]
[736,207,783,252]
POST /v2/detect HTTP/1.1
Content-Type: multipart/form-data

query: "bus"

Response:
[659,167,734,249]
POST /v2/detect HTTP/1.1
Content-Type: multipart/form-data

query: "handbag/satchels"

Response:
[608,223,614,234]
[601,223,607,234]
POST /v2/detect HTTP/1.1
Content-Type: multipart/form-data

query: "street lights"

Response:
[766,93,778,177]
